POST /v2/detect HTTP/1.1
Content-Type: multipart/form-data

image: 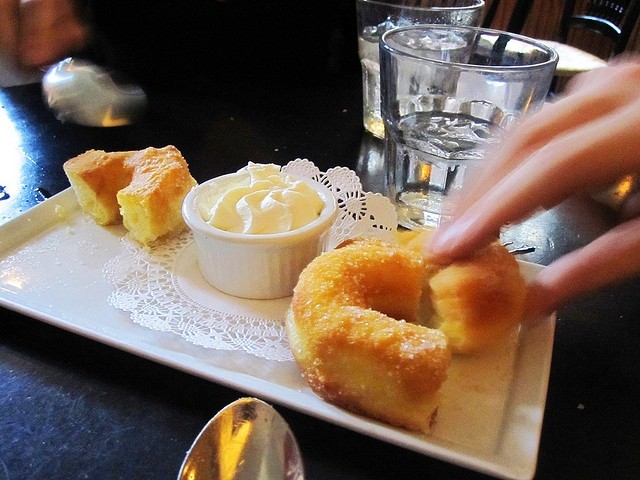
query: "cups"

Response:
[379,24,559,233]
[356,0,484,140]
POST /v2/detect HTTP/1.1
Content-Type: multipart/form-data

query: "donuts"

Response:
[287,239,528,434]
[64,146,200,245]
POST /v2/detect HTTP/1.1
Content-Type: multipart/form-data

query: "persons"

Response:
[423,52,638,330]
[2,1,89,67]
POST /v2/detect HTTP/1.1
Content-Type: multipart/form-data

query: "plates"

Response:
[0,186,557,480]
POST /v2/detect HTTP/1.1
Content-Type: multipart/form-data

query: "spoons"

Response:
[175,396,304,480]
